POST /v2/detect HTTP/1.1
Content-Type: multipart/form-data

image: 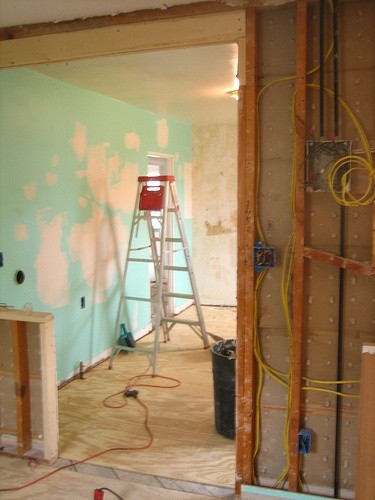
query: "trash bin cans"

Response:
[212,338,236,438]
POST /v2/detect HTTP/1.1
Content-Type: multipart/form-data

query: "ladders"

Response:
[108,175,211,377]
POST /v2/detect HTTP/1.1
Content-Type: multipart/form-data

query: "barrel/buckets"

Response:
[210,339,236,439]
